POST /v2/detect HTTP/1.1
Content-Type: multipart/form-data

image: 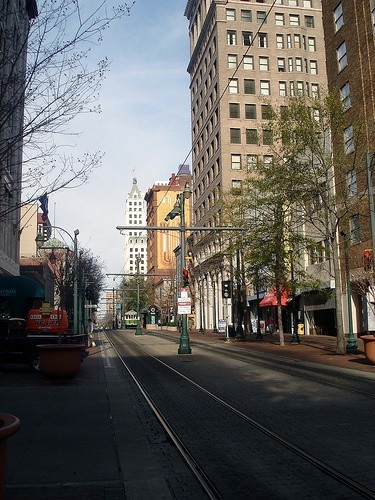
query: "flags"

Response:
[38,192,48,215]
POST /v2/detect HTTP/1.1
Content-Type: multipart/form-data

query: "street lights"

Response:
[287,249,301,345]
[253,266,264,340]
[135,254,144,335]
[174,181,193,354]
[121,275,127,331]
[34,225,79,341]
[340,227,359,350]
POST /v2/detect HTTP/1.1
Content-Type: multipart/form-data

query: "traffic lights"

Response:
[364,249,372,272]
[182,269,188,286]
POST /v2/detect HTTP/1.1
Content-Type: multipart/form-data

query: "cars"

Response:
[0,318,90,374]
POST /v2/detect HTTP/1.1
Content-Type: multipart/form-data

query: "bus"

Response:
[124,310,140,329]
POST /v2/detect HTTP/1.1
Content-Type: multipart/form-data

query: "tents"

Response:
[258,285,293,307]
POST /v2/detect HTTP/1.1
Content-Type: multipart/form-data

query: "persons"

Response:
[267,318,277,335]
